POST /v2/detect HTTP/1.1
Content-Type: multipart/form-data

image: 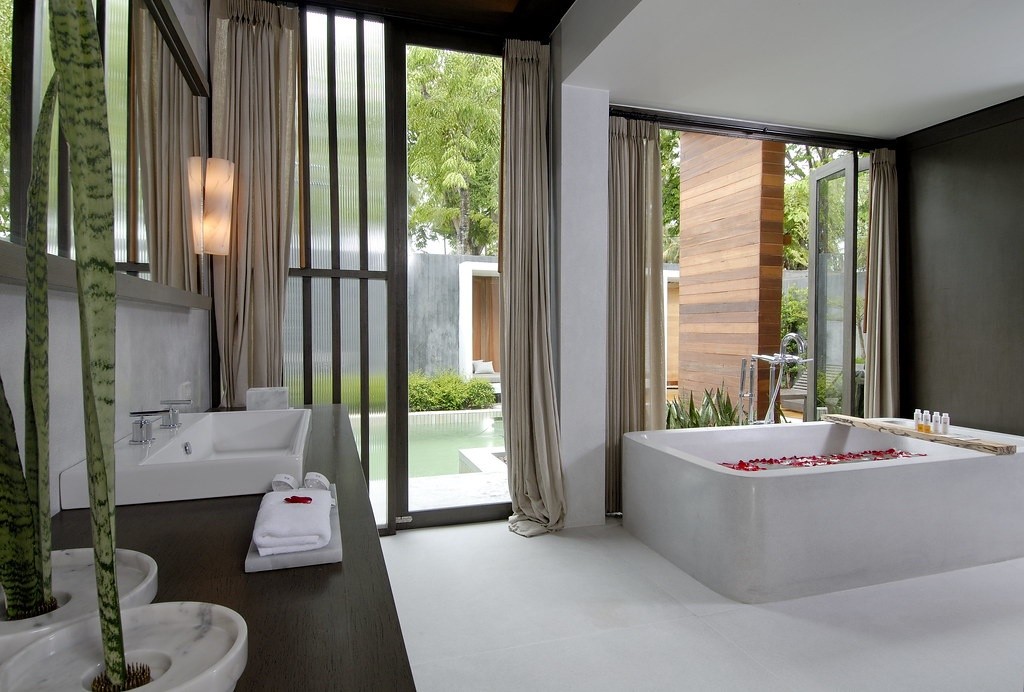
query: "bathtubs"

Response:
[621,417,1024,606]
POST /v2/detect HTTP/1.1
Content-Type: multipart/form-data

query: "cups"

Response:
[816,407,829,421]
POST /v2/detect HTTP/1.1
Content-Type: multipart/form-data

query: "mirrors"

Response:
[0,0,211,312]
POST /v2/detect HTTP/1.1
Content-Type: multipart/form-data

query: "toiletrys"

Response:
[913,409,950,436]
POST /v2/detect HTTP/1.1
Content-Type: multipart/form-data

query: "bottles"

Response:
[914,409,950,435]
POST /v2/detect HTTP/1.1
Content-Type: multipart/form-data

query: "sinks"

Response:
[138,409,312,505]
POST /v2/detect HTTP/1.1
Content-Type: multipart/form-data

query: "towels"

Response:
[252,489,332,558]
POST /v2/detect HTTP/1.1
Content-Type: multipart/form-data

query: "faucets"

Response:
[129,409,170,443]
[159,400,193,425]
[780,332,805,357]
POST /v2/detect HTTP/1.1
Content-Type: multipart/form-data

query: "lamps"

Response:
[203,158,235,257]
[186,155,203,256]
